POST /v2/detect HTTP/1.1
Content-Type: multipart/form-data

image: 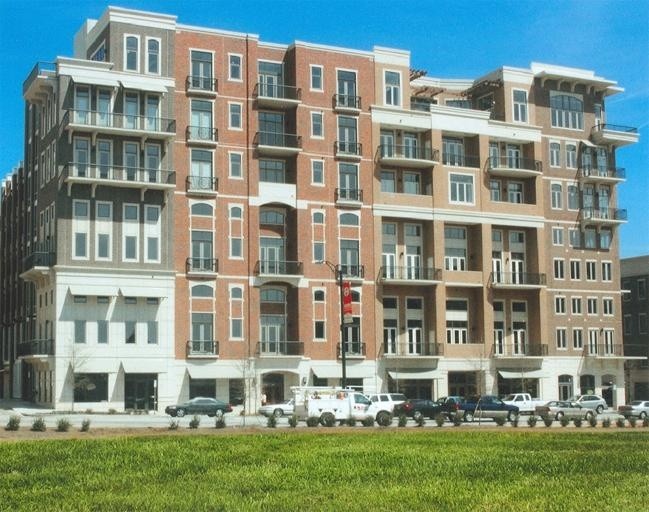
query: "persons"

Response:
[262,392,268,407]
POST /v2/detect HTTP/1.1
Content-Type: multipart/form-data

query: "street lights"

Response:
[321,260,348,390]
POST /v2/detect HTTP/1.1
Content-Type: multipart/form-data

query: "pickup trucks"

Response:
[501,393,549,415]
[445,395,520,422]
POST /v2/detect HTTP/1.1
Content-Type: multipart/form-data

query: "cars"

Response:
[258,397,294,418]
[535,401,598,422]
[289,386,467,427]
[164,396,233,418]
[564,395,609,414]
[618,401,649,420]
[127,392,156,409]
